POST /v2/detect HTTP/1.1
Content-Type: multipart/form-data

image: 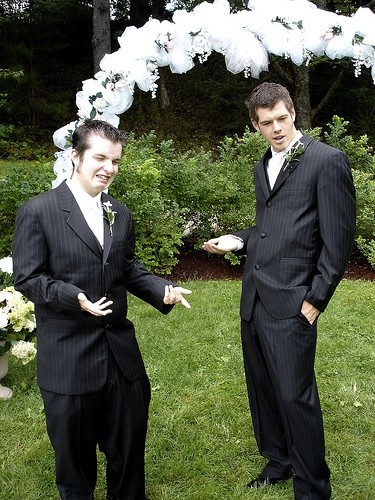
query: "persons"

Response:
[12,120,192,500]
[201,82,357,500]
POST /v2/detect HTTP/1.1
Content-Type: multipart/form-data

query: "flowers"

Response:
[281,137,305,172]
[49,0,375,190]
[100,199,117,237]
[0,257,37,365]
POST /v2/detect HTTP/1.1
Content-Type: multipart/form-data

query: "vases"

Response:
[0,354,14,400]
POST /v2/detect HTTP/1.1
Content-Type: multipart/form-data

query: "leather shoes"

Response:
[246,470,293,488]
